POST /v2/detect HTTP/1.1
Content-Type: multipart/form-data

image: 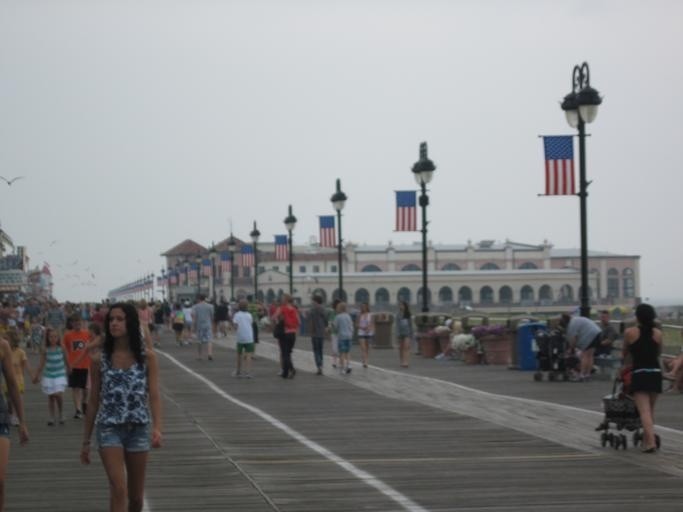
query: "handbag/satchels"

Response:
[271,320,285,338]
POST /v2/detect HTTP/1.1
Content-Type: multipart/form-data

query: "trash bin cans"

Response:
[415,312,452,355]
[506,315,540,370]
[373,313,395,348]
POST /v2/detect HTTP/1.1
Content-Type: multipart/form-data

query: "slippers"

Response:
[640,445,657,454]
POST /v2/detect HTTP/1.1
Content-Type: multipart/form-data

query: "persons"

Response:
[62,313,92,419]
[18,416,26,422]
[1,292,308,350]
[622,304,662,452]
[0,336,30,512]
[329,302,354,376]
[192,295,214,360]
[558,313,602,382]
[662,354,682,394]
[305,293,331,375]
[433,319,476,362]
[354,301,375,368]
[0,331,36,426]
[393,299,412,369]
[271,293,301,378]
[594,368,623,432]
[593,310,617,356]
[662,357,680,374]
[79,302,164,512]
[33,326,72,425]
[231,302,254,379]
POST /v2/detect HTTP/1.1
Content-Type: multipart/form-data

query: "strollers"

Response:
[527,329,578,386]
[592,359,661,451]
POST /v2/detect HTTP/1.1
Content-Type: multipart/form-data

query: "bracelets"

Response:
[27,368,33,375]
[82,439,91,445]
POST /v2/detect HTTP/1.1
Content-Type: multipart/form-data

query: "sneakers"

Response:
[57,416,66,424]
[287,369,295,378]
[82,403,87,414]
[344,365,352,374]
[46,418,55,425]
[75,410,81,417]
[278,369,288,377]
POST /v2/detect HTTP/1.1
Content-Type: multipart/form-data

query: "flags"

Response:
[275,234,287,261]
[395,190,416,231]
[542,135,574,195]
[108,259,211,297]
[220,255,231,271]
[241,246,253,267]
[319,215,336,247]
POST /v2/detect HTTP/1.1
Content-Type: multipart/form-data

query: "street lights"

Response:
[223,234,238,301]
[559,58,605,323]
[282,205,298,307]
[207,244,218,307]
[329,176,348,314]
[250,221,262,304]
[194,250,205,305]
[410,139,436,314]
